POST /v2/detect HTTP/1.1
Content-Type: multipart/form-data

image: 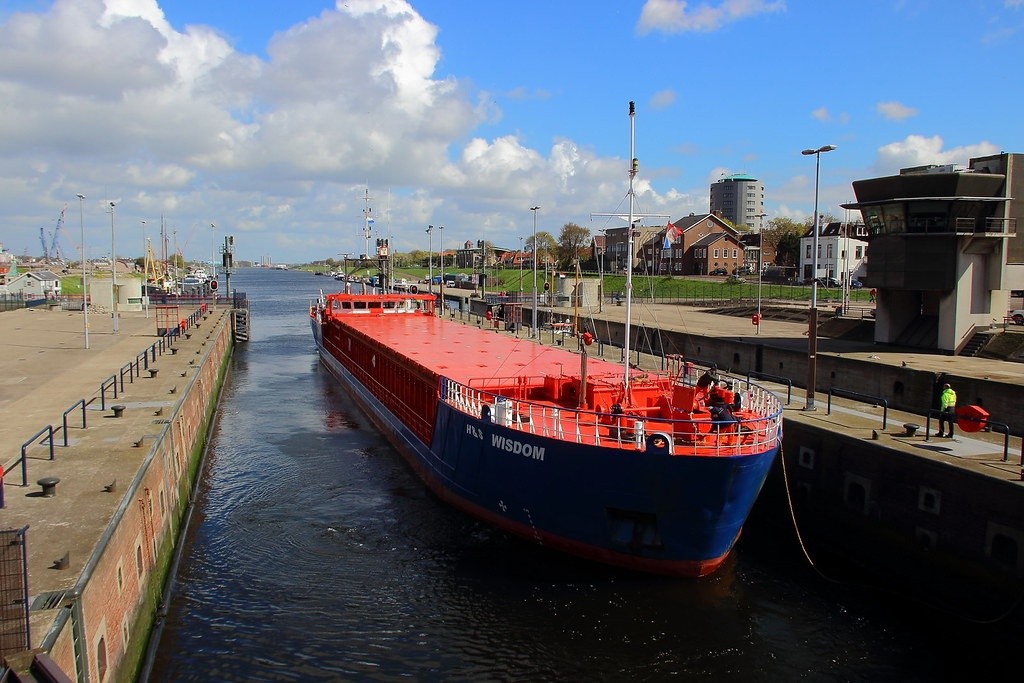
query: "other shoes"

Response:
[935,432,943,437]
[944,433,953,438]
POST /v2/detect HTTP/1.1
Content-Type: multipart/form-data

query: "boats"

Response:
[142,262,213,296]
[314,269,408,292]
[309,278,784,584]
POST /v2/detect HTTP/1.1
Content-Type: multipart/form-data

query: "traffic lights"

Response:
[209,280,219,291]
[411,285,418,294]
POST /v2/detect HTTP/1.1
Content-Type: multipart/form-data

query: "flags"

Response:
[663,222,683,249]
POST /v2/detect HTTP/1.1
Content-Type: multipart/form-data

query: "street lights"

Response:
[736,241,746,281]
[140,219,149,319]
[210,223,216,310]
[110,201,119,333]
[425,224,434,294]
[172,230,178,306]
[754,213,767,334]
[597,228,605,313]
[802,143,838,412]
[519,236,524,296]
[544,233,548,307]
[76,193,90,349]
[615,242,624,277]
[530,206,542,339]
[438,226,444,316]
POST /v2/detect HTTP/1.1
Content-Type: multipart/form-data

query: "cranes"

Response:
[40,203,71,268]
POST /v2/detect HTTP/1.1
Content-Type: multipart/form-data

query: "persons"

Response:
[935,383,956,438]
[870,288,876,303]
[695,367,734,446]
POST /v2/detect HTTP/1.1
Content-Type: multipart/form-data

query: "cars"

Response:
[726,274,746,284]
[840,279,863,290]
[446,280,455,288]
[732,261,777,277]
[817,276,841,288]
[1010,308,1024,326]
[709,267,728,276]
